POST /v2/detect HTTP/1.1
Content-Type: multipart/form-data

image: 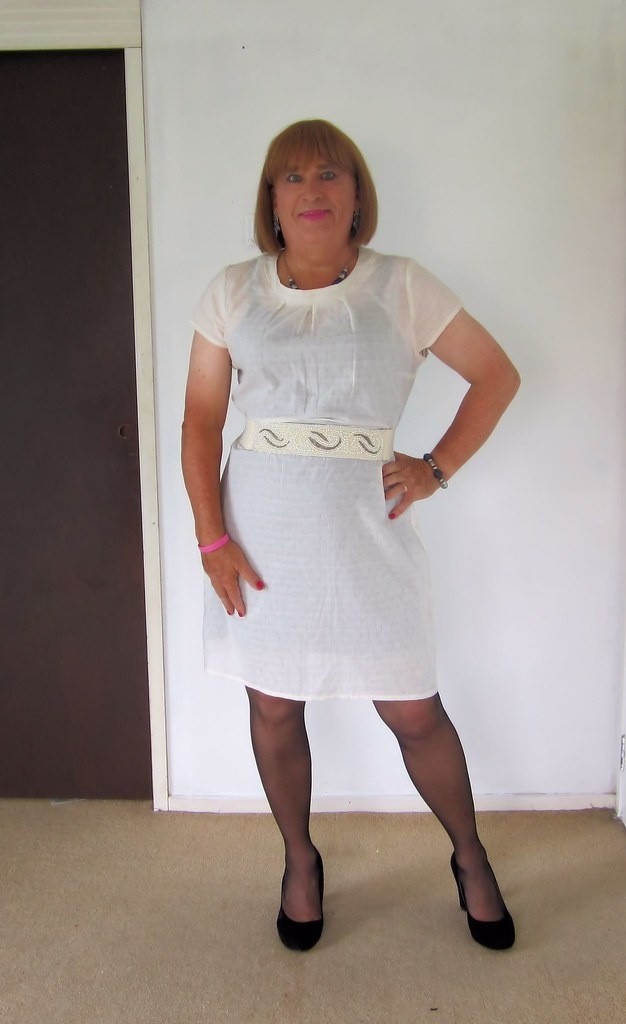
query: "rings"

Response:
[402,483,408,491]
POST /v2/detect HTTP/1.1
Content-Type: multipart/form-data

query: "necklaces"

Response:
[283,252,348,289]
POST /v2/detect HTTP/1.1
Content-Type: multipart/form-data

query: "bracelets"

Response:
[423,453,448,489]
[198,534,230,553]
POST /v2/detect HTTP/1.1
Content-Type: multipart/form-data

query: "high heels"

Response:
[277,845,324,951]
[449,850,516,950]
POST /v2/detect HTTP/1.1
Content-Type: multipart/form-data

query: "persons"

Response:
[181,119,521,950]
